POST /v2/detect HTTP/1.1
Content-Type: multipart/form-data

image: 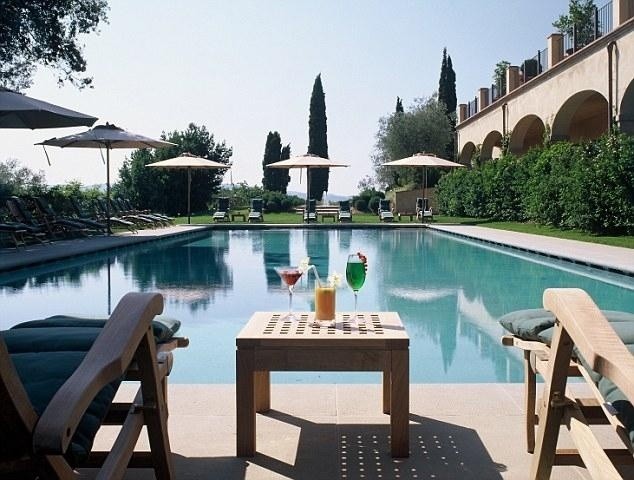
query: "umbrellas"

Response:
[32,121,178,233]
[144,151,231,224]
[388,231,463,305]
[374,149,465,223]
[0,86,99,128]
[264,152,351,223]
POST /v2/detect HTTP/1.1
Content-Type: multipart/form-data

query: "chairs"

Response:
[377,199,394,223]
[0,292,188,480]
[302,199,317,223]
[338,200,352,223]
[211,197,230,222]
[247,199,264,223]
[415,197,433,224]
[498,287,634,480]
[0,195,176,254]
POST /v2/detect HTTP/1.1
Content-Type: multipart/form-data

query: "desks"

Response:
[397,212,414,221]
[230,213,246,222]
[317,212,337,222]
[234,311,411,458]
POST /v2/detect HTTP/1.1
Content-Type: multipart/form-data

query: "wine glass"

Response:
[344,252,369,321]
[273,265,308,322]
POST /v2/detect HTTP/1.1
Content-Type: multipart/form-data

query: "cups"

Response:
[313,279,336,328]
